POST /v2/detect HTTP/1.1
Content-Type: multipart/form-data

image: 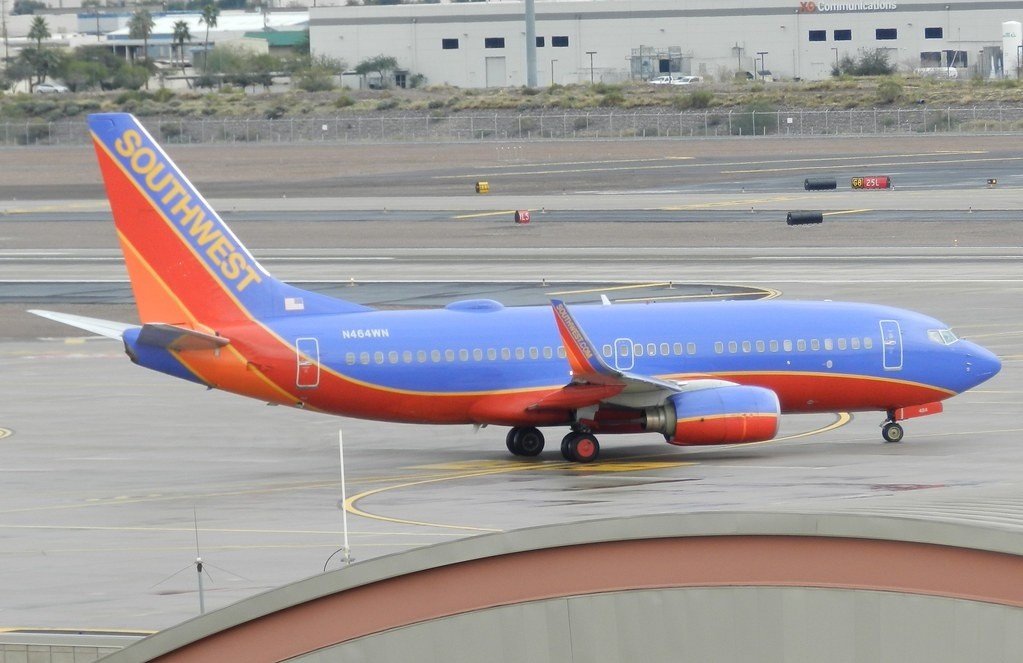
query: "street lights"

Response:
[831,47,839,67]
[754,59,761,81]
[757,51,768,79]
[1016,45,1023,81]
[586,52,597,86]
[552,59,558,88]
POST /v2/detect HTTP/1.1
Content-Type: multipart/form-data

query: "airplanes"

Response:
[84,111,1003,465]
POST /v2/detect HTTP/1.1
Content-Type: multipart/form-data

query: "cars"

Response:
[650,76,673,84]
[36,82,68,93]
[671,76,703,85]
